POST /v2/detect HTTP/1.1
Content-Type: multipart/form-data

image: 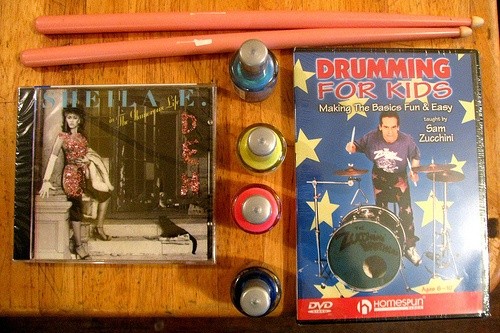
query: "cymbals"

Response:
[411,165,456,171]
[427,170,466,183]
[333,168,367,176]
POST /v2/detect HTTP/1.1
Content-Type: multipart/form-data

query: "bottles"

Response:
[227,38,279,102]
[228,261,283,317]
[231,183,283,235]
[236,122,287,177]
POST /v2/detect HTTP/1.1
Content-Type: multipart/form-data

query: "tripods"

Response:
[298,176,355,300]
[415,176,466,279]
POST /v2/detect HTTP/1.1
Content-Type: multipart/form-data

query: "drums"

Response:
[326,205,408,292]
